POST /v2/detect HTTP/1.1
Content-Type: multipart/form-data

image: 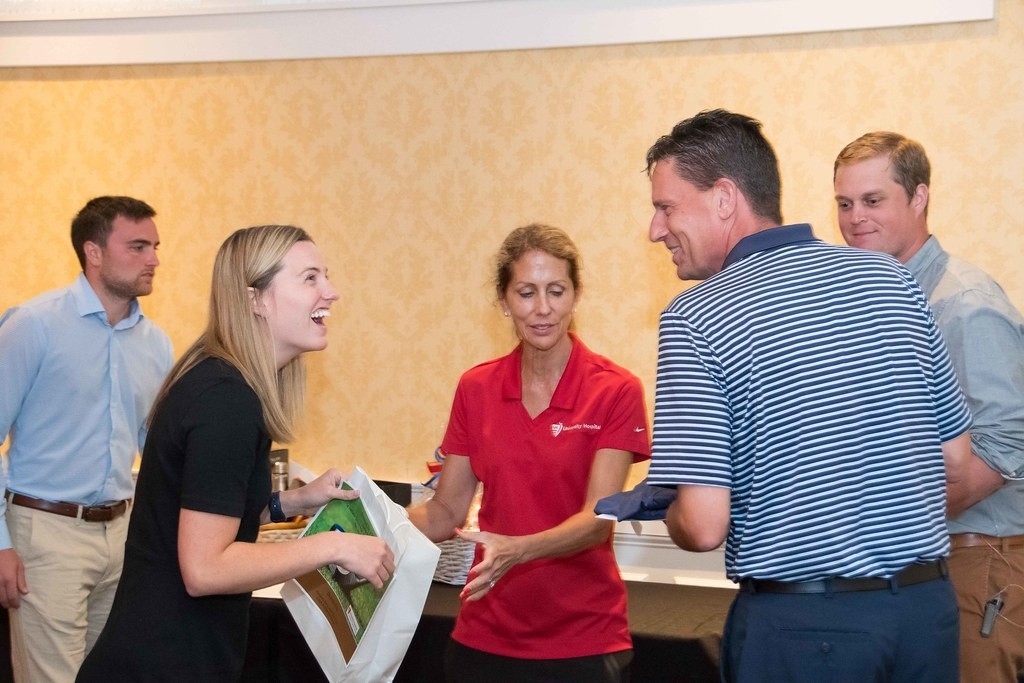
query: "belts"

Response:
[950,532,1023,550]
[4,490,131,523]
[740,558,949,594]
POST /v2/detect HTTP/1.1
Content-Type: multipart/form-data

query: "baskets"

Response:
[255,513,318,544]
[432,529,481,586]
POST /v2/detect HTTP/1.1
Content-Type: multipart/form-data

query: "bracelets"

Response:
[268,491,286,523]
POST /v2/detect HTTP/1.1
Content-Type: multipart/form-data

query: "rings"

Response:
[489,581,495,588]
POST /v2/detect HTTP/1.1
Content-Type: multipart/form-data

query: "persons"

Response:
[833,131,1023,683]
[407,222,651,682]
[76,225,396,683]
[644,110,973,682]
[0,197,174,683]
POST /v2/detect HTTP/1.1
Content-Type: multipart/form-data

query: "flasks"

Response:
[273,461,289,492]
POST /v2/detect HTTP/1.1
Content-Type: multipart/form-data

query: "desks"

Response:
[408,548,737,640]
